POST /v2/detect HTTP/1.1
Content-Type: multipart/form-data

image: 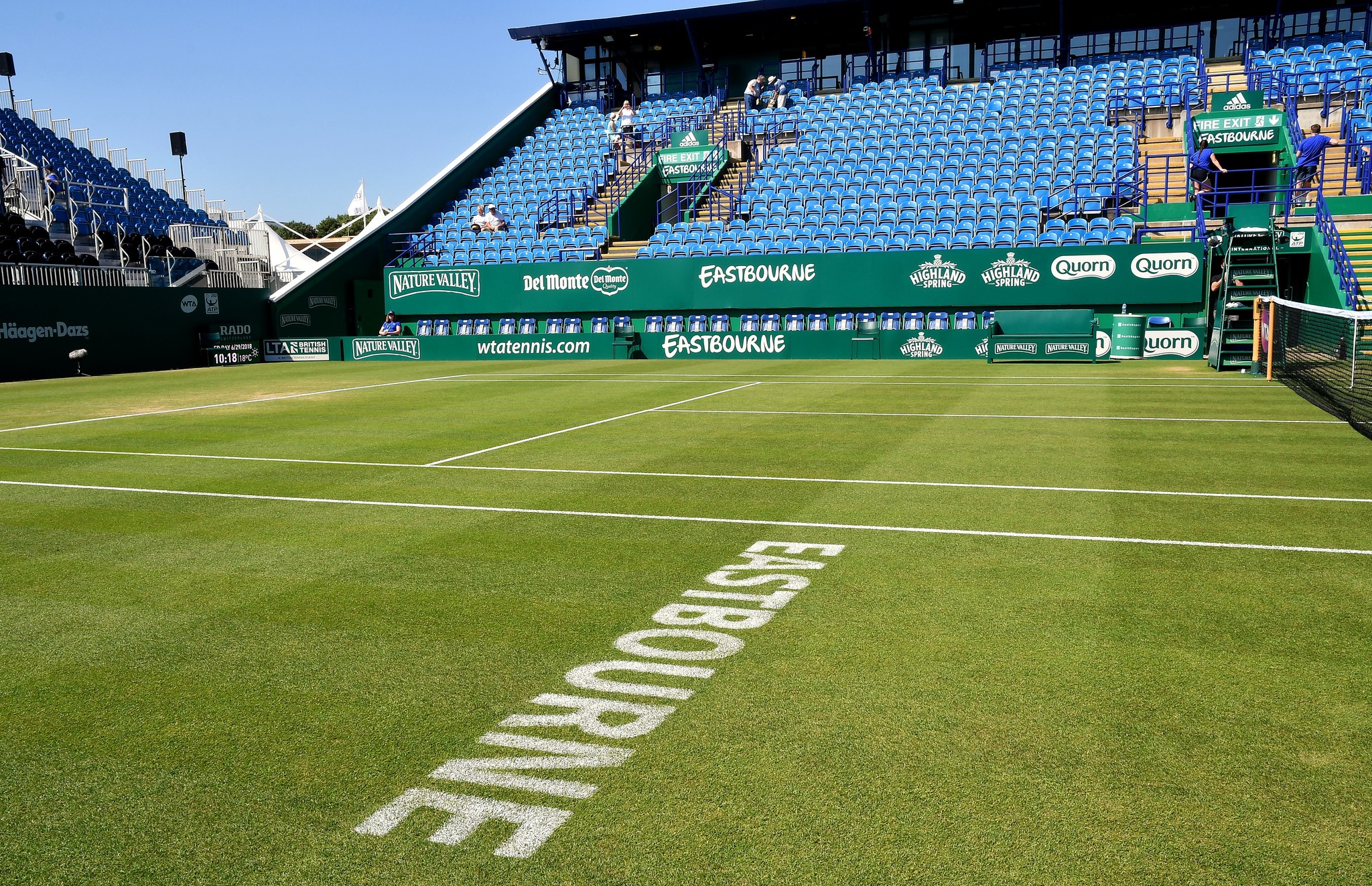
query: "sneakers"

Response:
[1291,204,1303,209]
[1189,195,1193,202]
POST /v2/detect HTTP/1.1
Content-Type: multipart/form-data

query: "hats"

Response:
[387,311,395,315]
[41,165,54,172]
[768,75,776,85]
[609,113,619,121]
[488,204,495,209]
[470,224,480,230]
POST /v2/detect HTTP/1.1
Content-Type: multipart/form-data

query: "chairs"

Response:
[498,317,515,334]
[1147,316,1172,328]
[644,316,663,332]
[545,317,563,333]
[612,315,630,333]
[760,312,993,332]
[664,316,683,332]
[416,319,432,336]
[393,30,1372,268]
[563,317,581,333]
[518,317,536,333]
[473,318,490,335]
[740,314,759,331]
[710,314,729,331]
[456,318,472,335]
[688,314,707,332]
[433,319,449,336]
[851,321,880,360]
[0,106,251,288]
[612,325,635,360]
[591,317,608,333]
[1182,316,1208,351]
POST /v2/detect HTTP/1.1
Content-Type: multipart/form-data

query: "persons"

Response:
[472,204,487,231]
[768,76,788,109]
[0,155,8,215]
[481,204,505,232]
[1210,258,1245,352]
[607,113,621,150]
[745,75,766,113]
[1361,146,1372,173]
[606,74,623,91]
[39,164,60,211]
[1292,123,1339,207]
[1189,140,1228,208]
[378,310,400,337]
[618,100,636,140]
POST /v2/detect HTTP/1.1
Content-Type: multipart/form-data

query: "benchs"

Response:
[987,309,1098,364]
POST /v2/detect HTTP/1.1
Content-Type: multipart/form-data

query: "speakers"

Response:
[169,132,188,155]
[0,51,16,76]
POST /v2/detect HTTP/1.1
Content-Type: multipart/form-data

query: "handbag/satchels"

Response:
[768,92,778,108]
[617,138,633,149]
[494,225,503,232]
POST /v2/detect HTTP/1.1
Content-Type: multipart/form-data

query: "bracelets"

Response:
[4,177,6,179]
